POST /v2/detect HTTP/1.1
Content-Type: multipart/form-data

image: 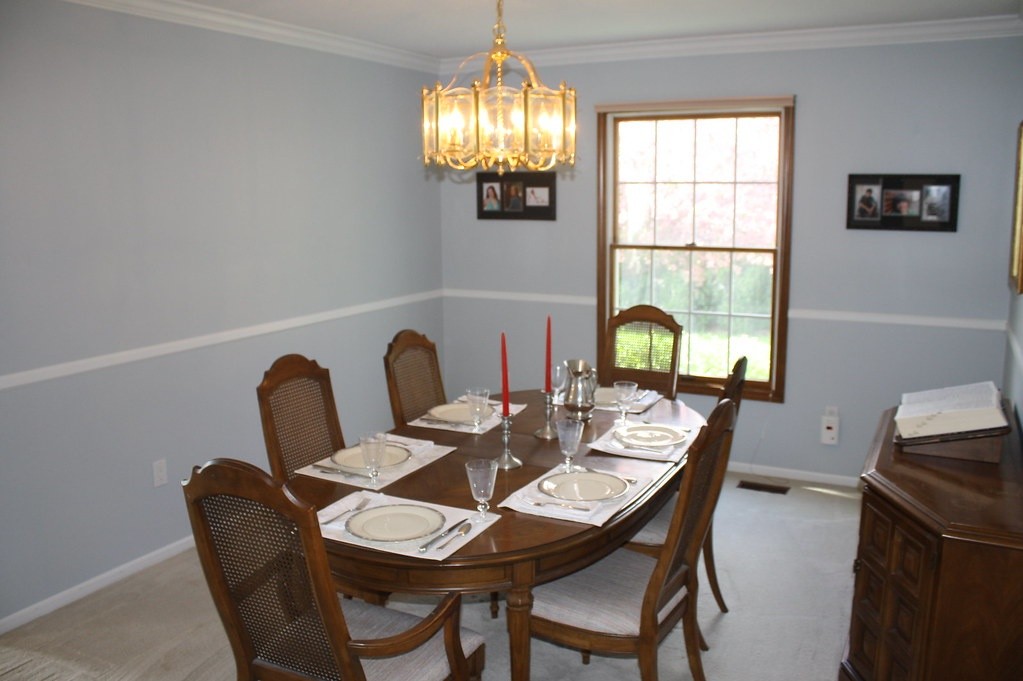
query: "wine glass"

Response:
[550,365,567,411]
[555,419,585,473]
[465,387,491,432]
[613,380,638,433]
[465,459,498,523]
[360,433,388,486]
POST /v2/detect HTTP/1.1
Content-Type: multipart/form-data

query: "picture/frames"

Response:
[477,172,556,220]
[1008,120,1022,296]
[846,174,960,232]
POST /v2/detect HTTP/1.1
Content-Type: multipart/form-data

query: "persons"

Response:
[891,194,916,214]
[858,189,877,217]
[484,186,501,211]
[506,186,522,210]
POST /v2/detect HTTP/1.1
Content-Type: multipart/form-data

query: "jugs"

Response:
[564,359,597,420]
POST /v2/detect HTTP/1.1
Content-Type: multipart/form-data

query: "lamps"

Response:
[422,1,576,175]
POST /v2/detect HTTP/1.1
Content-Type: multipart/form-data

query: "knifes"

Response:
[642,421,692,432]
[420,418,464,424]
[585,467,637,485]
[312,463,372,480]
[418,518,469,552]
[632,389,650,404]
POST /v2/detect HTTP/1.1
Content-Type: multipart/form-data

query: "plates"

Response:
[593,387,637,405]
[427,402,495,425]
[537,470,629,502]
[613,424,687,447]
[345,503,446,542]
[331,444,411,469]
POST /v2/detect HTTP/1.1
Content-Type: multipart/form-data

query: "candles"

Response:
[546,316,551,393]
[501,333,509,417]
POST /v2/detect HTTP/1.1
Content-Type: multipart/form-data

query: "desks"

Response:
[286,388,707,681]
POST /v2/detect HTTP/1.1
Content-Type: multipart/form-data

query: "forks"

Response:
[521,497,592,512]
[456,399,502,406]
[320,497,371,526]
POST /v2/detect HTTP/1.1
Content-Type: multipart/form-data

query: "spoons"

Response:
[319,469,356,478]
[437,523,472,550]
[427,421,462,427]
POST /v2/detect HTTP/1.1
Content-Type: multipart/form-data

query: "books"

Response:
[893,381,1008,440]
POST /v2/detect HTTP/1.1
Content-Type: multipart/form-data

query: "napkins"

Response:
[496,463,653,528]
[293,432,457,491]
[406,395,527,435]
[315,490,502,561]
[552,387,664,413]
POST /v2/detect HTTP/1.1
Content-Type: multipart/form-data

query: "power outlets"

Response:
[153,460,168,487]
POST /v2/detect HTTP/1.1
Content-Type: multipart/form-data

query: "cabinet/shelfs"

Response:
[838,398,1023,681]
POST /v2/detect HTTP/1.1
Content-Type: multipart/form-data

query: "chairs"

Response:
[530,398,736,681]
[384,329,447,427]
[181,458,485,681]
[598,304,683,400]
[624,356,747,651]
[256,353,392,623]
